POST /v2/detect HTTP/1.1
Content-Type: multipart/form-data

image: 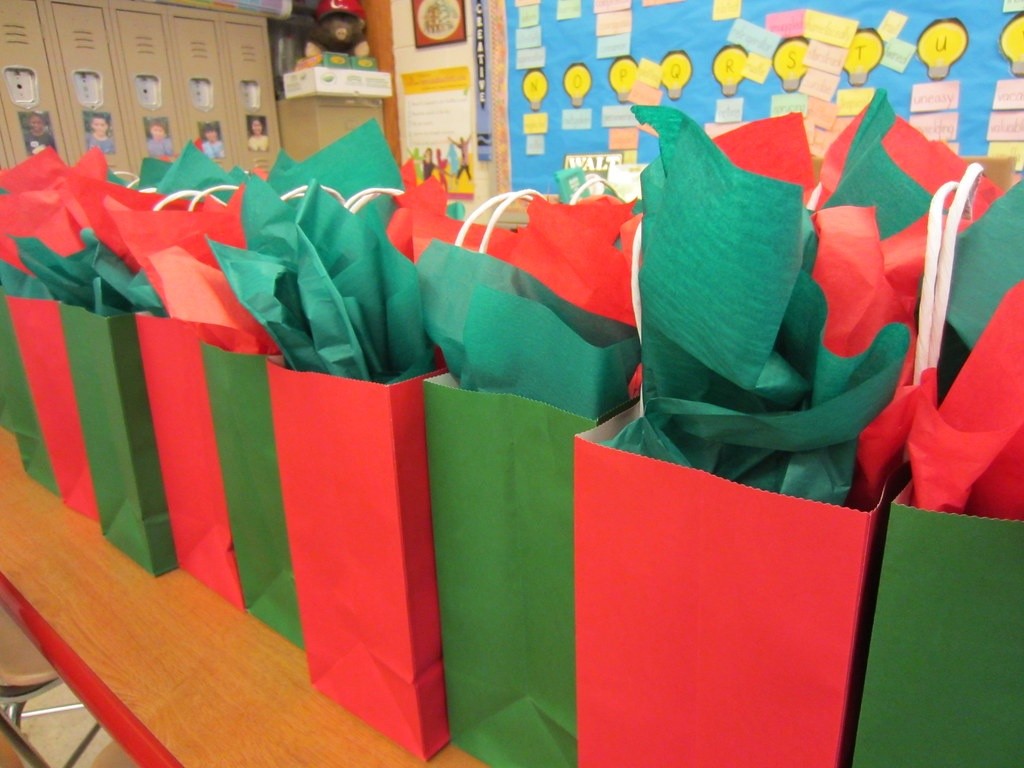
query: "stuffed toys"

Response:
[303,0,370,58]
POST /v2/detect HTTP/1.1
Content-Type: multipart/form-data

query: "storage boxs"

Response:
[282,53,393,101]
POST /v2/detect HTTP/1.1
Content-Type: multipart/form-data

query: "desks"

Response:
[0,423,495,768]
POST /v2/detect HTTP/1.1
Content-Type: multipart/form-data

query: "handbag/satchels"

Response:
[266,187,532,762]
[852,162,1024,768]
[0,172,380,652]
[423,174,640,768]
[574,181,960,768]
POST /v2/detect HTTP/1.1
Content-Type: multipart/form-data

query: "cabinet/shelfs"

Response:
[0,0,281,180]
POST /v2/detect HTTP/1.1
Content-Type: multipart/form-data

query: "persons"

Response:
[202,124,224,159]
[85,114,117,154]
[23,112,57,156]
[146,120,174,157]
[248,119,270,152]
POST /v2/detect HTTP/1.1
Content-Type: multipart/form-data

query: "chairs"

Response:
[0,606,63,729]
